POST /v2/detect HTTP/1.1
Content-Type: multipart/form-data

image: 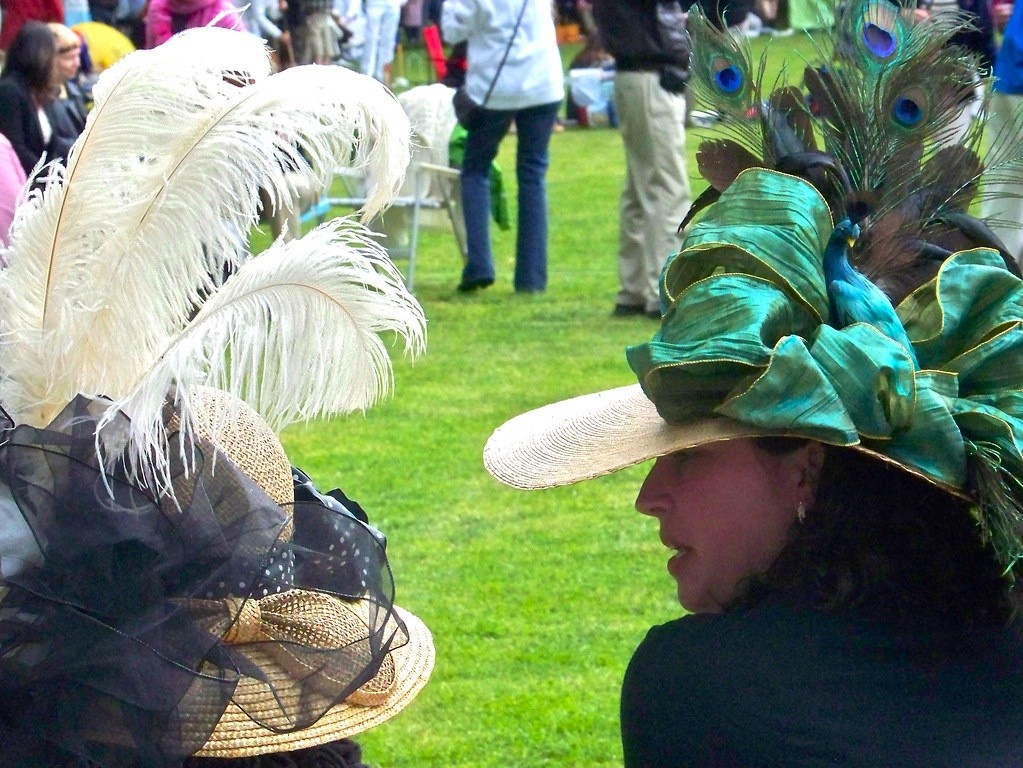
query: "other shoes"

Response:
[612,304,662,319]
[458,278,490,291]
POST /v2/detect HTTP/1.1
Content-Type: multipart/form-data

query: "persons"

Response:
[481,269,1023,768]
[439,0,566,293]
[587,1,694,317]
[0,399,436,768]
[1,0,1023,318]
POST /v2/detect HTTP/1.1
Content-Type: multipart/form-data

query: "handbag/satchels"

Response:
[453,87,480,132]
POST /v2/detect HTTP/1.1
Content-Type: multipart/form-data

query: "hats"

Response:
[483,0,1023,491]
[0,26,436,768]
[47,23,81,52]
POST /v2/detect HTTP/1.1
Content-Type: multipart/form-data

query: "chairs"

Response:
[306,82,473,299]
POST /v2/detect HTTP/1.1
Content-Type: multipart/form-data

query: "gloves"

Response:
[302,14,344,63]
